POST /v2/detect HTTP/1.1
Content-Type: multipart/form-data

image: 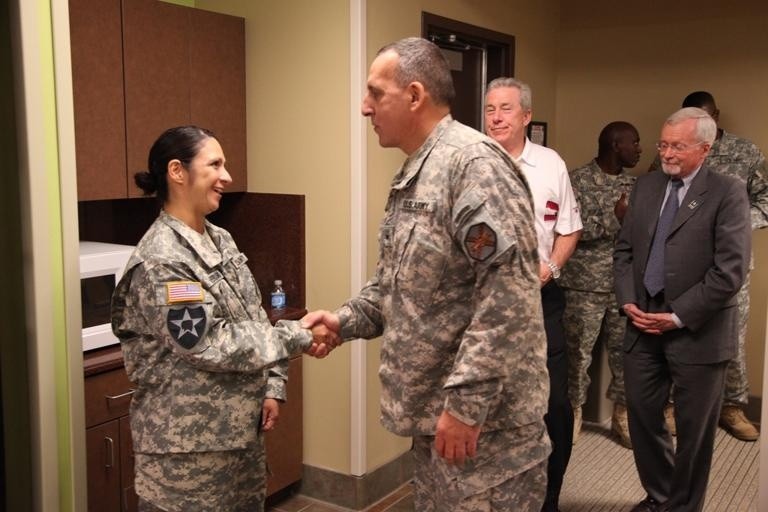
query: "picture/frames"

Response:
[528,121,548,148]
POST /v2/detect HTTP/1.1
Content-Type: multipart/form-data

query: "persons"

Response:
[109,122,343,510]
[294,36,555,510]
[648,89,767,441]
[474,73,586,510]
[560,115,643,451]
[604,107,752,512]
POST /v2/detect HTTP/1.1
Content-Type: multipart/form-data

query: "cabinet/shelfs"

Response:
[264,309,307,498]
[66,2,245,203]
[85,366,139,512]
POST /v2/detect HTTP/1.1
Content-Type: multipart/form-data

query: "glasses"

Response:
[655,140,709,153]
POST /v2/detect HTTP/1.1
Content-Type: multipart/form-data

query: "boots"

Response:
[609,402,635,451]
[569,404,585,445]
[720,405,760,443]
[663,398,678,436]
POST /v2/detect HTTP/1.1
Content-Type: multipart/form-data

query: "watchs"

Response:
[546,260,562,282]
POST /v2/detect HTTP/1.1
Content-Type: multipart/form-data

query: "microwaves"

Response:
[77,240,140,353]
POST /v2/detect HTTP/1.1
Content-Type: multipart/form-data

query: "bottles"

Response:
[268,279,285,317]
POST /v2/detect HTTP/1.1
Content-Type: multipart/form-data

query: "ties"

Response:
[638,177,689,301]
